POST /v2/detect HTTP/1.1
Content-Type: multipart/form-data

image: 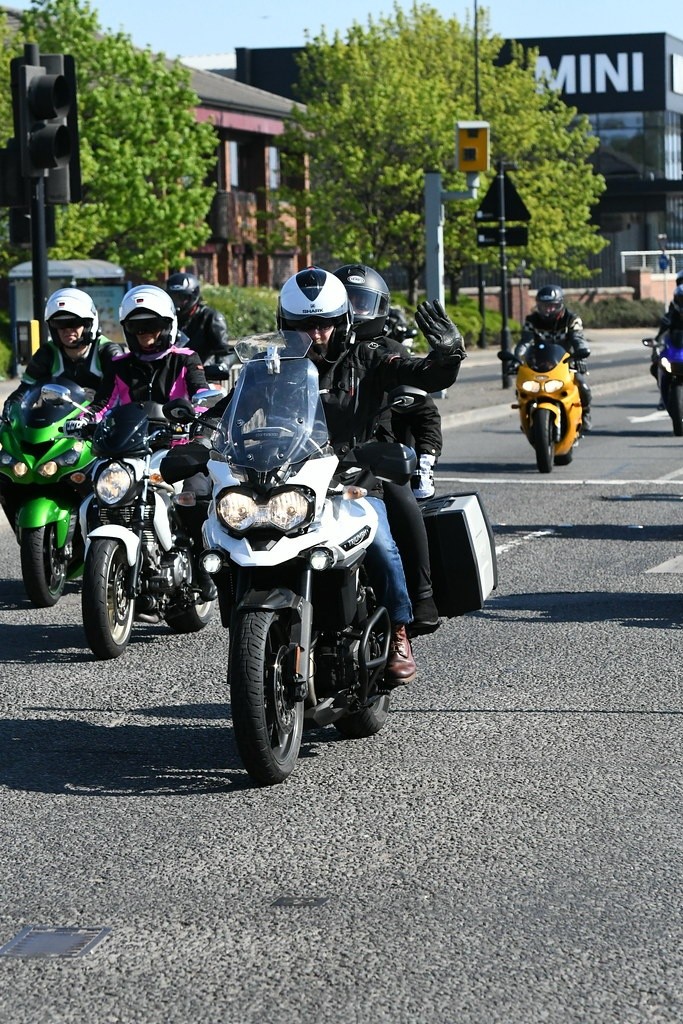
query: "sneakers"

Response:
[580,414,593,431]
[411,597,438,626]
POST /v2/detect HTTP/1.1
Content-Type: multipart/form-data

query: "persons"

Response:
[63,285,218,600]
[650,269,683,409]
[189,266,468,684]
[332,263,443,627]
[2,287,126,421]
[166,272,236,375]
[510,285,593,431]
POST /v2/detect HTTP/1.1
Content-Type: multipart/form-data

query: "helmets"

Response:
[118,285,177,345]
[276,268,356,350]
[45,288,99,339]
[674,269,683,295]
[536,285,564,318]
[166,273,200,314]
[332,265,391,339]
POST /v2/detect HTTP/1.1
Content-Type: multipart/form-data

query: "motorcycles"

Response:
[642,327,683,436]
[0,332,500,786]
[498,343,592,475]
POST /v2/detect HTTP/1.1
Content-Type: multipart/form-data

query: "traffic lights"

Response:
[11,52,83,204]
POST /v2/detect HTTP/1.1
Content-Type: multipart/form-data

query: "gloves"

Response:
[415,300,467,359]
[206,364,229,380]
[2,402,10,424]
[166,443,210,477]
[64,418,88,439]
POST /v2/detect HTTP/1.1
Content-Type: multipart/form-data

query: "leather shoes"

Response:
[197,565,219,600]
[386,626,417,683]
